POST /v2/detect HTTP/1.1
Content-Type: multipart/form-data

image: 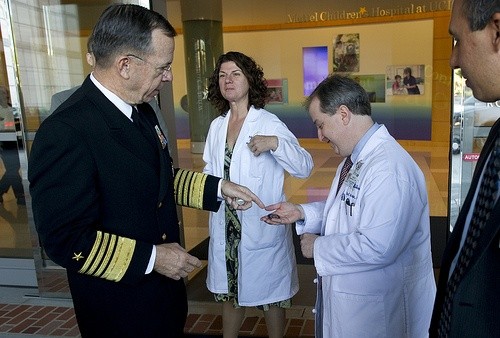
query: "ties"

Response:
[335,154,353,198]
[131,107,161,161]
[437,139,499,338]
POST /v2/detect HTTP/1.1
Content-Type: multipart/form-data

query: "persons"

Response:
[50,27,171,158]
[260,74,436,338]
[203,51,314,338]
[334,40,345,64]
[391,67,420,96]
[429,0,500,338]
[28,3,265,338]
[0,88,26,206]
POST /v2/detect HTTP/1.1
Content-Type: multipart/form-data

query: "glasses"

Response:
[126,54,171,76]
